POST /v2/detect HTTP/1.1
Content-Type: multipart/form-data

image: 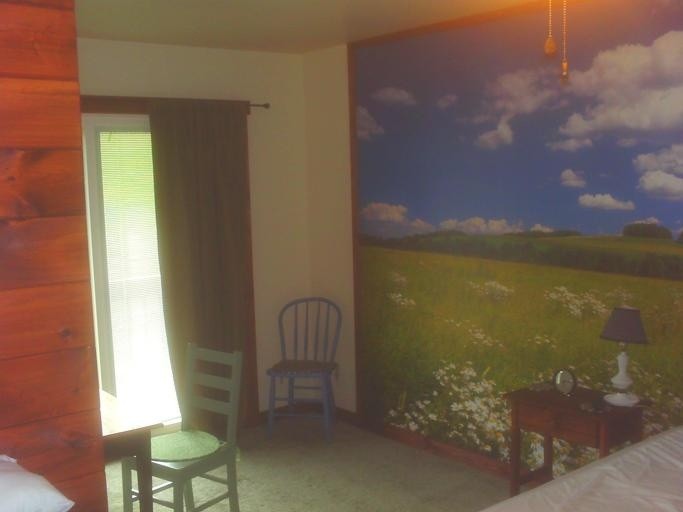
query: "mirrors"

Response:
[553,368,578,396]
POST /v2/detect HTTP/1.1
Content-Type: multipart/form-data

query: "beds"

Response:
[480,424,683,512]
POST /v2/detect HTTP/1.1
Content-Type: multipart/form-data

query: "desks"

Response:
[99,389,161,511]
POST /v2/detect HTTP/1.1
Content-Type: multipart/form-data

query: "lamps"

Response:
[599,308,648,407]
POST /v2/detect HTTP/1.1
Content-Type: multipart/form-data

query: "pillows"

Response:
[0,454,75,512]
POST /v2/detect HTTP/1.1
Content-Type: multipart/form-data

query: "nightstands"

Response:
[509,380,641,496]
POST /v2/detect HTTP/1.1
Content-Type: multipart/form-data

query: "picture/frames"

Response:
[346,0,683,479]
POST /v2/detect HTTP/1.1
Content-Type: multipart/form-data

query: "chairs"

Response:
[121,340,243,512]
[266,297,342,437]
